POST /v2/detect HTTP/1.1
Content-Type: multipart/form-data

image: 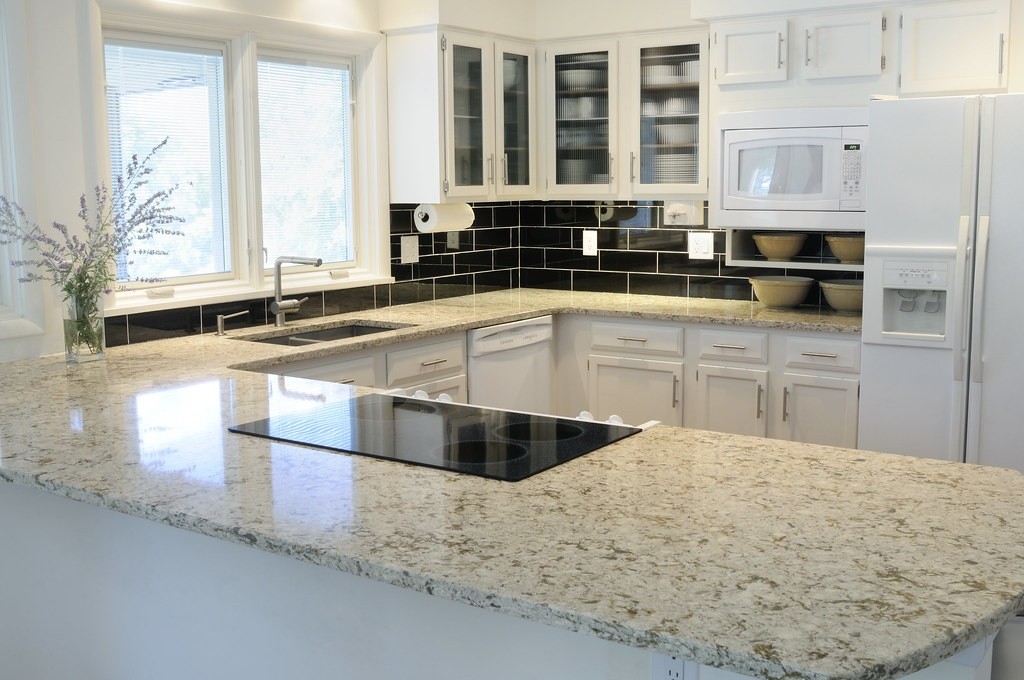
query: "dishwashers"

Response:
[467,314,553,417]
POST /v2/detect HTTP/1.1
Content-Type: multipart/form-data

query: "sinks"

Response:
[224,319,423,347]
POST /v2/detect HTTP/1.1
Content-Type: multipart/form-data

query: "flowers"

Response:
[1,136,192,349]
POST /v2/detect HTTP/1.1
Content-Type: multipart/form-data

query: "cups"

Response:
[504,60,521,90]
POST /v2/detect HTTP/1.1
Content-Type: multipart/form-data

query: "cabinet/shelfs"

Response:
[541,31,710,202]
[707,9,1015,98]
[256,330,471,405]
[583,318,860,450]
[385,29,537,202]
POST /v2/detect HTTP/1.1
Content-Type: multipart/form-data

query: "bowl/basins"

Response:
[653,125,696,144]
[557,70,604,90]
[752,232,808,262]
[824,234,865,265]
[818,278,862,316]
[749,275,815,311]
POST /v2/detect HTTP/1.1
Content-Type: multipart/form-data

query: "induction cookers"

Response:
[227,390,642,482]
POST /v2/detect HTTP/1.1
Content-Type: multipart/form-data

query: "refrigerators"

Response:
[856,93,1024,476]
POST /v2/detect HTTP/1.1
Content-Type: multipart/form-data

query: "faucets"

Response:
[270,256,322,327]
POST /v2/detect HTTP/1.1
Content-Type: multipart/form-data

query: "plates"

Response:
[556,123,608,146]
[642,97,699,115]
[641,61,699,84]
[558,159,608,184]
[651,153,697,182]
[568,54,608,61]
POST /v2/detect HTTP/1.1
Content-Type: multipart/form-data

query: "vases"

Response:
[62,316,106,362]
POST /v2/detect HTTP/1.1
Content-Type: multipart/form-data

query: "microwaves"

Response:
[722,127,865,213]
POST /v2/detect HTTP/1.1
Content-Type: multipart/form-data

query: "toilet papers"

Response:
[414,202,475,234]
[594,201,637,221]
